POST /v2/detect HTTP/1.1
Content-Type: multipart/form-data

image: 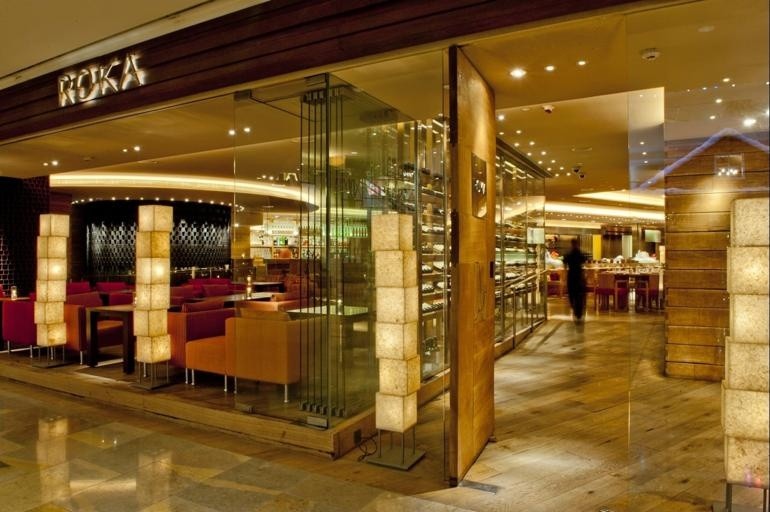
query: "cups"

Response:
[245,274,254,300]
[11,286,17,299]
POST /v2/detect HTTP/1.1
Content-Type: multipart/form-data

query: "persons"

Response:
[563,239,588,321]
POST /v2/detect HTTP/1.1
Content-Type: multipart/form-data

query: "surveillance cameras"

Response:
[580,173,584,178]
[573,168,579,173]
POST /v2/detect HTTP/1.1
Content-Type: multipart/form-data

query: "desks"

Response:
[286,304,369,370]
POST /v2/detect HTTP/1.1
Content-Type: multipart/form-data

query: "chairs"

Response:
[0,277,328,403]
[594,275,660,312]
